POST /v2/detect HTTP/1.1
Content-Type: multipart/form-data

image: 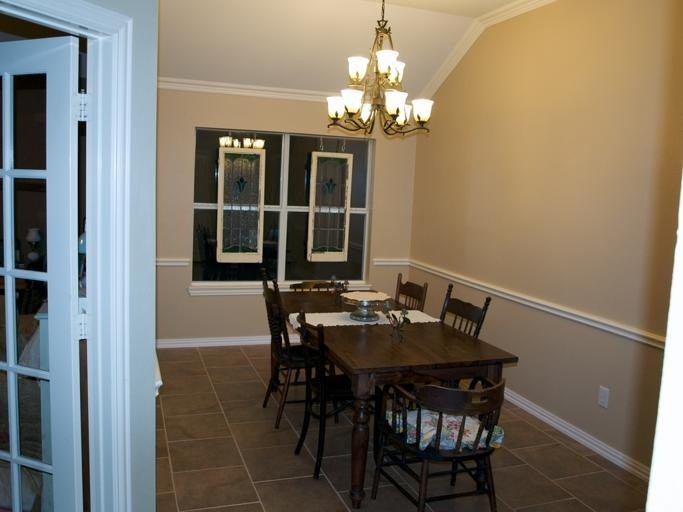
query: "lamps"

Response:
[219,131,265,149]
[326,0,434,136]
[27,228,42,261]
